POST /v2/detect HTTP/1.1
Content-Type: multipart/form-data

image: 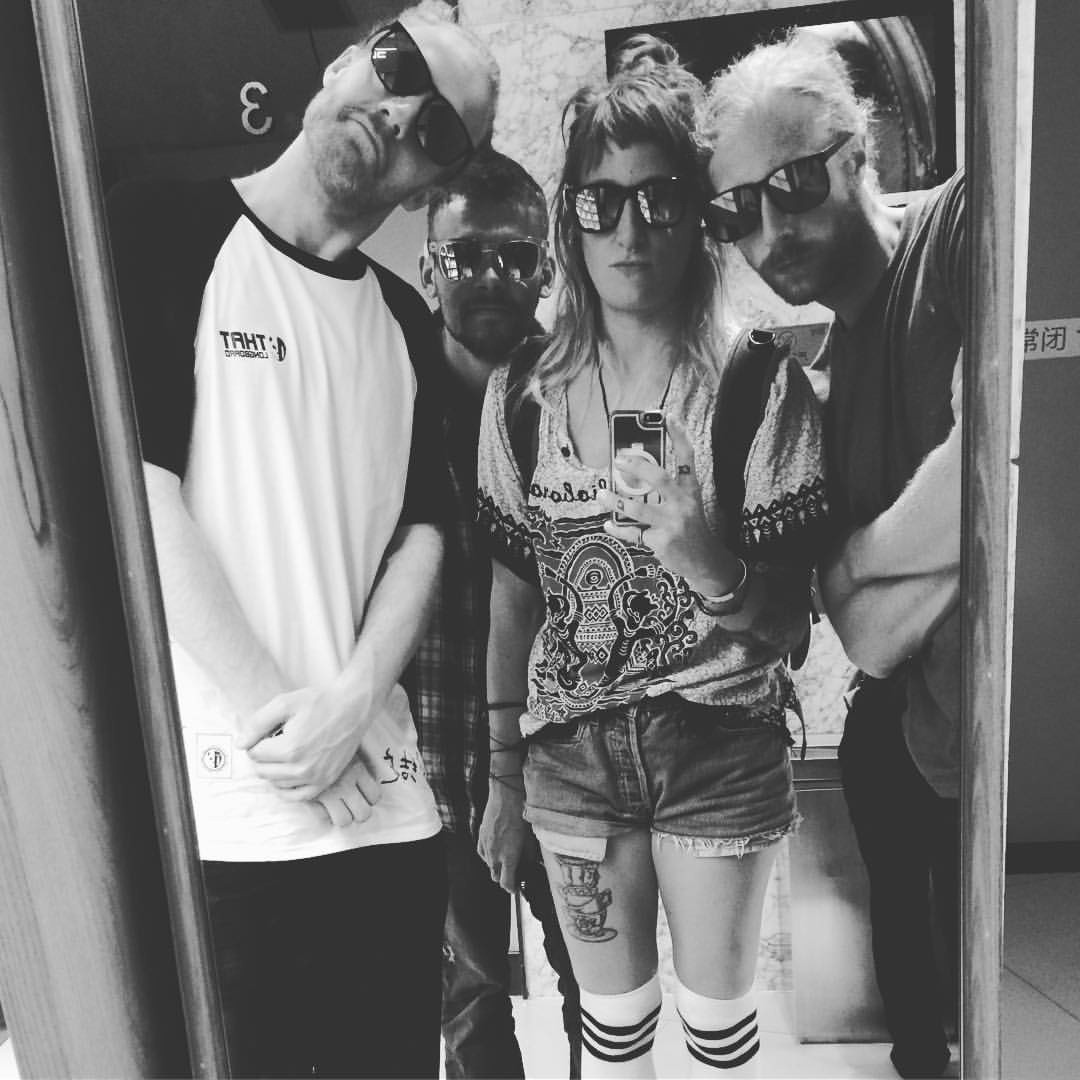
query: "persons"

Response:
[126,8,500,1078]
[410,141,555,1080]
[695,36,965,1080]
[475,55,833,1080]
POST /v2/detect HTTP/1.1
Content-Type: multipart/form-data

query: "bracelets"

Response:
[692,595,747,619]
[707,559,747,604]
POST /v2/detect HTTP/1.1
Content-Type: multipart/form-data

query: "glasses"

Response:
[573,176,699,232]
[429,237,541,282]
[368,20,474,167]
[701,136,849,243]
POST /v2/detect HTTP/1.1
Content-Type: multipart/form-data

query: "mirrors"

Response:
[28,2,1032,1080]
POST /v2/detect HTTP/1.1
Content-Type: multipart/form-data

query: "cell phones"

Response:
[609,409,666,528]
